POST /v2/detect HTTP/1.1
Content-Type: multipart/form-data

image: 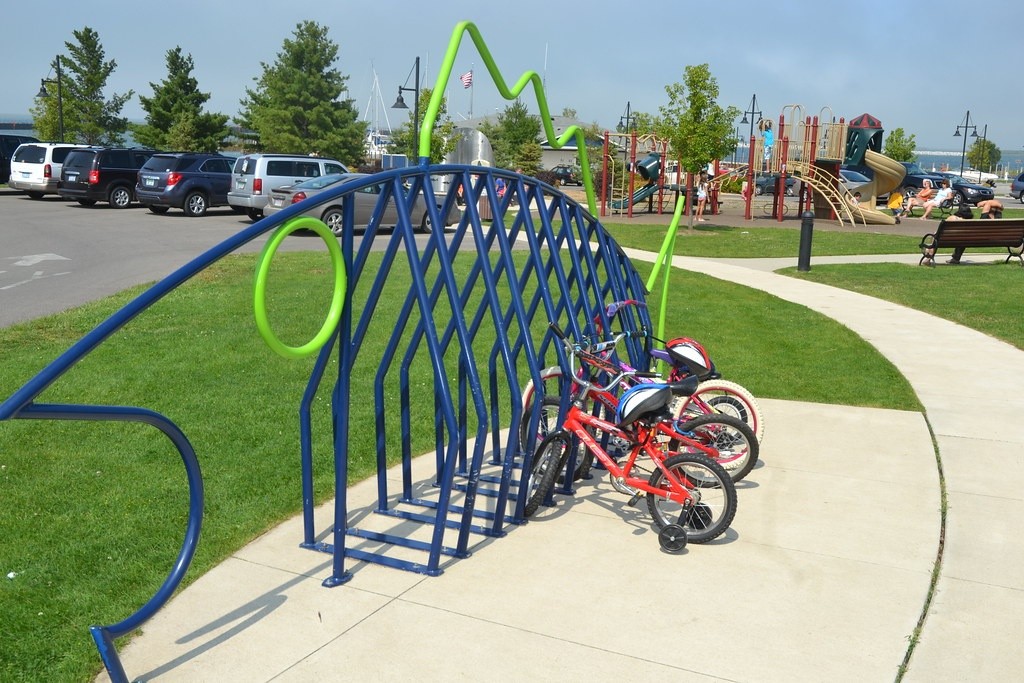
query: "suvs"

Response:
[7,142,89,199]
[226,154,349,221]
[134,149,237,218]
[56,147,156,208]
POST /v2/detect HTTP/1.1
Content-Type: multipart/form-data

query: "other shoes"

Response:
[698,218,705,221]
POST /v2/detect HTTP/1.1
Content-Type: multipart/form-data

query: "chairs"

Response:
[306,167,314,176]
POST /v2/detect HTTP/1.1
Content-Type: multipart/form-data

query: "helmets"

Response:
[665,337,711,377]
[617,384,671,428]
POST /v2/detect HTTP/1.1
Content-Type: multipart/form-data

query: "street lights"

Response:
[953,110,977,177]
[36,54,64,143]
[617,101,637,160]
[740,95,763,135]
[390,57,420,162]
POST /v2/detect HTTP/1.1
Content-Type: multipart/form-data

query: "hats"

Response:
[922,179,933,188]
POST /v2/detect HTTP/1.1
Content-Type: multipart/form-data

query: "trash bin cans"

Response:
[479,189,500,222]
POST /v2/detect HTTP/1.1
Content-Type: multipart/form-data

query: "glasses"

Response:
[942,183,947,184]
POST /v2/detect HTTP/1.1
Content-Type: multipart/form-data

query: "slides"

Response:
[607,183,661,209]
[844,149,907,225]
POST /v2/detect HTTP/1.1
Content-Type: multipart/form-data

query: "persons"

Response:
[759,119,775,173]
[902,179,933,218]
[923,203,973,265]
[495,168,523,206]
[850,192,865,209]
[887,188,905,224]
[918,178,952,220]
[947,200,1004,263]
[693,173,711,222]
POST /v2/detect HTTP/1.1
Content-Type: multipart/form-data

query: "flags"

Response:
[460,70,472,89]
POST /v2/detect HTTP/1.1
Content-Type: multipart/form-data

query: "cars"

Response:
[263,171,462,238]
[1010,171,1024,202]
[543,160,994,204]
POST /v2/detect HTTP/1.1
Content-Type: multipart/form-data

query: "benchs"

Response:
[919,218,1024,268]
[902,189,957,218]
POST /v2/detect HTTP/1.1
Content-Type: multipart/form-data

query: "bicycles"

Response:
[521,299,765,551]
